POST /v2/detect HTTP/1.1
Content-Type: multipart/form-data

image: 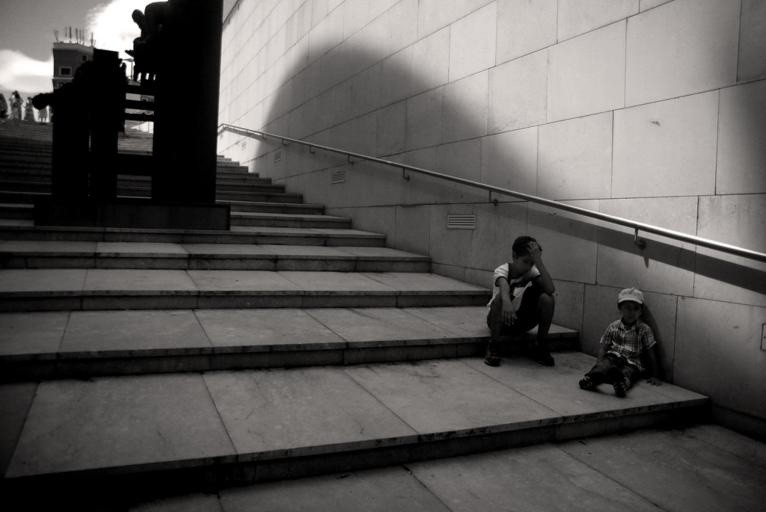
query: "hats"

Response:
[617,287,644,305]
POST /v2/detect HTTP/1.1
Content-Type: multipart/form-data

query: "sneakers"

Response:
[484,345,501,367]
[579,373,599,390]
[533,348,555,367]
[612,376,627,397]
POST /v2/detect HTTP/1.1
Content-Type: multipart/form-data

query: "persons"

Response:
[38,107,48,124]
[578,287,663,399]
[7,90,24,122]
[22,96,37,123]
[481,234,557,369]
